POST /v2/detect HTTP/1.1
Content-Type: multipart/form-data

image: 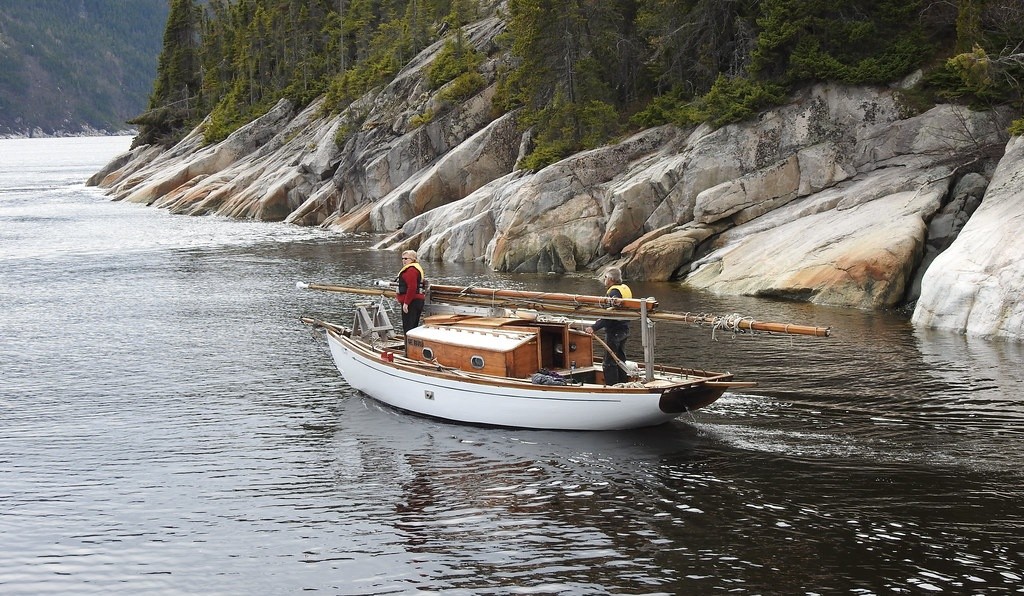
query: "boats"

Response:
[296,282,831,431]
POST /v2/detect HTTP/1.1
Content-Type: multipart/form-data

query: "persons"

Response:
[584,266,632,387]
[395,249,425,354]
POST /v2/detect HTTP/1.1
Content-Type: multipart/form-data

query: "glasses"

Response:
[402,258,411,260]
